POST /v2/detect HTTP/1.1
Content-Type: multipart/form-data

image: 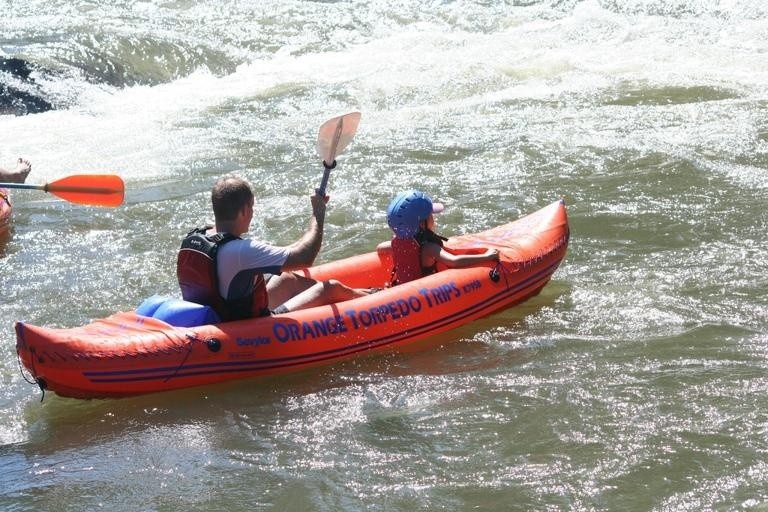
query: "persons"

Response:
[0,157,32,186]
[174,176,373,322]
[386,189,500,286]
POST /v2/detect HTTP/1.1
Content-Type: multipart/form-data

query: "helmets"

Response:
[387,190,444,239]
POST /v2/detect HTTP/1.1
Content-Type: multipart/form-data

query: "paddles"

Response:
[316,113,362,194]
[0,175,124,207]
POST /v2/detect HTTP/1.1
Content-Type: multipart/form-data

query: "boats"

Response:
[0,183,16,249]
[12,194,575,404]
